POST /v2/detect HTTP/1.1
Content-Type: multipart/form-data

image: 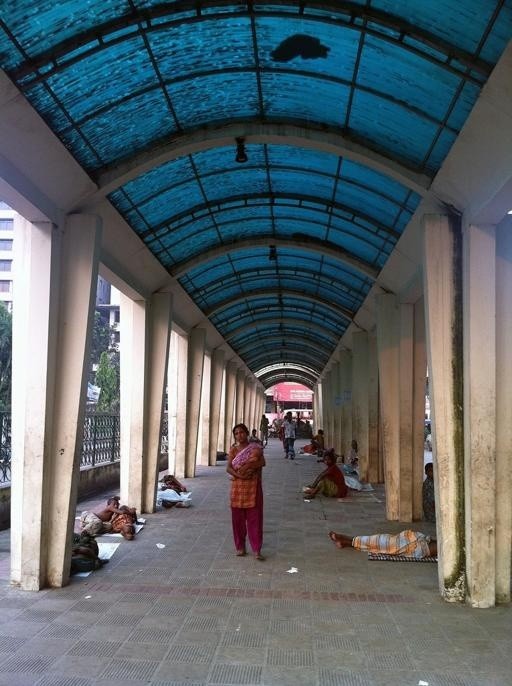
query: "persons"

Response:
[344,438,359,477]
[159,474,191,509]
[260,414,269,445]
[247,428,260,442]
[69,495,139,575]
[279,416,287,460]
[229,446,261,482]
[226,424,265,560]
[422,462,436,522]
[283,411,297,461]
[328,529,436,559]
[303,429,325,453]
[301,450,347,500]
[266,416,314,439]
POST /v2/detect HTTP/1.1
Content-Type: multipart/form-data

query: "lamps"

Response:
[235,135,247,163]
[268,243,277,260]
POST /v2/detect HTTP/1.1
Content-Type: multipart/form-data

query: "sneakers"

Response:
[254,551,266,561]
[284,455,295,460]
[235,550,247,557]
